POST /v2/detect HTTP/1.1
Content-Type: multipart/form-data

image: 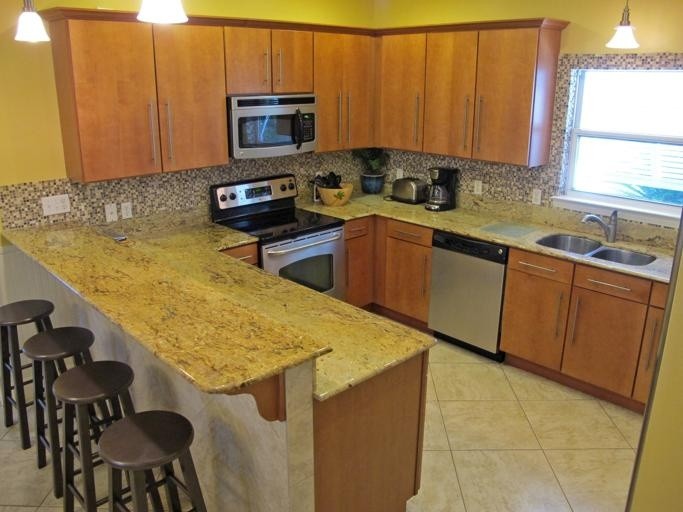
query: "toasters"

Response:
[390,177,428,205]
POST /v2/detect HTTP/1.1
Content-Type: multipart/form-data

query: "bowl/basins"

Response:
[317,183,354,206]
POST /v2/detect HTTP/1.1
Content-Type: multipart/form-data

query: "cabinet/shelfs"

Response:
[631,280,670,416]
[421,27,561,169]
[223,23,313,95]
[499,248,652,400]
[312,30,375,153]
[375,32,427,154]
[373,218,433,339]
[346,218,372,310]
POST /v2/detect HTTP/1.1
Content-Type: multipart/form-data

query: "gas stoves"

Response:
[210,176,350,243]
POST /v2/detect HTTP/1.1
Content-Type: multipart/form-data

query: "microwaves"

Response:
[260,226,350,302]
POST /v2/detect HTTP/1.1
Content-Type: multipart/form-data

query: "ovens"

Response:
[227,92,318,160]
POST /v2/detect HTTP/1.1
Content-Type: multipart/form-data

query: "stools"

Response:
[0,299,66,451]
[97,410,207,512]
[22,326,95,499]
[51,361,135,512]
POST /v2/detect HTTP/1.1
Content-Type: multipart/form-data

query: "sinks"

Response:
[585,245,657,267]
[534,232,603,257]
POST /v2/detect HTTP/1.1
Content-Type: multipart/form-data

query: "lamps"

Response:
[135,0,189,27]
[13,1,53,45]
[49,19,229,183]
[605,0,640,50]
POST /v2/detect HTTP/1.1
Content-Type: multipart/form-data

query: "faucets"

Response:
[579,208,619,242]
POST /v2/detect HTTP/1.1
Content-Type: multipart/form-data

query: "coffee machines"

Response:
[425,167,459,212]
